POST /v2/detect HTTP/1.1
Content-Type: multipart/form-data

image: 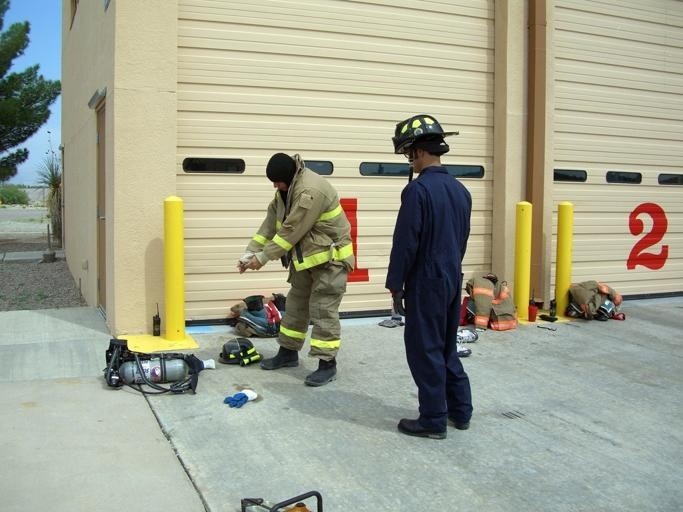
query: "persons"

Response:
[237,153,354,386]
[385,115,473,440]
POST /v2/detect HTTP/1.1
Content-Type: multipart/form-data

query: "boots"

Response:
[260,344,300,370]
[304,355,338,387]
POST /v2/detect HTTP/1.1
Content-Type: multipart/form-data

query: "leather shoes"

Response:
[397,409,449,441]
[444,401,473,430]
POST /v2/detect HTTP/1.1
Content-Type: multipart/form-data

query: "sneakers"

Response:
[453,328,479,344]
[449,342,471,357]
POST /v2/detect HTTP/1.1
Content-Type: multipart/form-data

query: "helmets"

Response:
[217,336,265,367]
[391,112,447,155]
